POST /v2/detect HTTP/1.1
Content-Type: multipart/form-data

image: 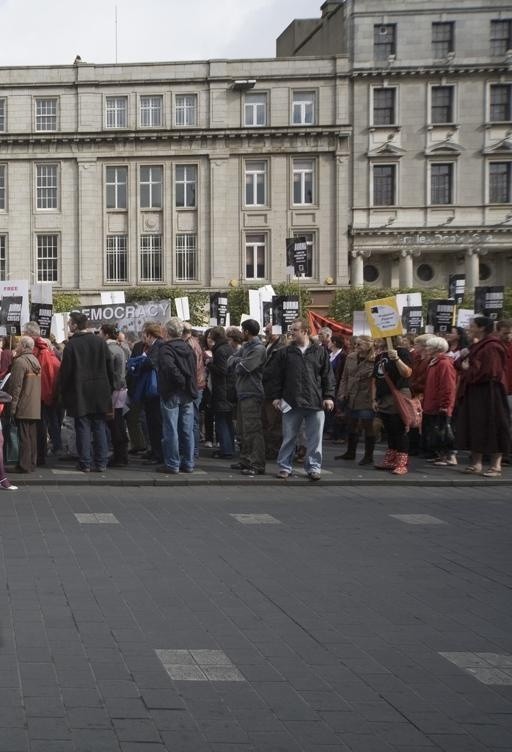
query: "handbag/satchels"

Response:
[199,387,215,417]
[397,397,423,435]
[427,413,455,450]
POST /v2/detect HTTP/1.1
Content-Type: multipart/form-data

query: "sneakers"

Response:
[204,440,212,448]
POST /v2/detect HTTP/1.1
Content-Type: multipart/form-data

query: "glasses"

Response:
[354,343,363,347]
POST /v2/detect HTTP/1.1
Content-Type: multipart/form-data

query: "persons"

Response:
[1,306,512,490]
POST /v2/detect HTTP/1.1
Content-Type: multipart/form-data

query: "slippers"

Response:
[426,457,502,477]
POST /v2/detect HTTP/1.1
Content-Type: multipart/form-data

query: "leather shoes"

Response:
[129,447,193,474]
[80,440,129,472]
[0,478,18,490]
[211,449,322,480]
[5,452,47,473]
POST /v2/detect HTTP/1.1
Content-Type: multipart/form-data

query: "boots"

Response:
[376,448,397,470]
[392,452,408,473]
[359,436,375,466]
[334,433,358,460]
